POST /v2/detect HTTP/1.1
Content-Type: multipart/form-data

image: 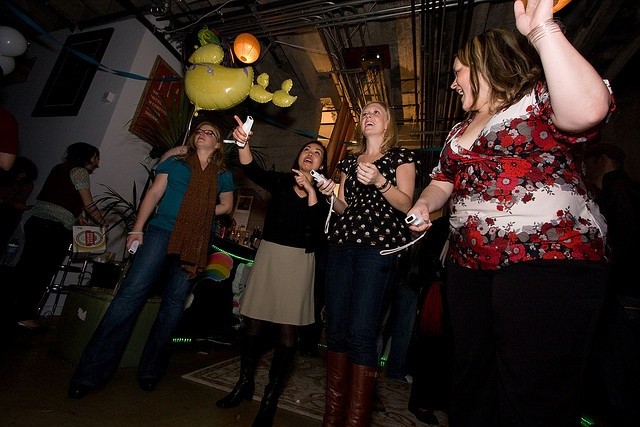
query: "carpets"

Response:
[181,342,450,427]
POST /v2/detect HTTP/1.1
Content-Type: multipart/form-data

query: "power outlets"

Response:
[102,89,115,106]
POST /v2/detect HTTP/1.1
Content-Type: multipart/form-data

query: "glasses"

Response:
[195,128,218,140]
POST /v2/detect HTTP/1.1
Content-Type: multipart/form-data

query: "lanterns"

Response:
[234,32,262,64]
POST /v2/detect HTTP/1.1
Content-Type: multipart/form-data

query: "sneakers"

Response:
[18,319,46,331]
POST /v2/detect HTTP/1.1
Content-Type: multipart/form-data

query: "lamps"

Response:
[0,25,33,77]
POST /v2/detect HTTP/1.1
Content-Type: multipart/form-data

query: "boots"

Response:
[343,362,383,427]
[250,341,296,426]
[215,332,262,408]
[321,349,351,426]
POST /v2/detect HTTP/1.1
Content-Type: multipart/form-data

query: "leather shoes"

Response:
[408,397,440,426]
[72,381,90,400]
[137,377,158,393]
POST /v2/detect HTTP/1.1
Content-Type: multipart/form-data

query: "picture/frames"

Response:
[29,26,115,119]
[128,55,195,155]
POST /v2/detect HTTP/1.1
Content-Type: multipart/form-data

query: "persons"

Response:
[1,106,19,173]
[68,119,239,400]
[213,115,333,427]
[0,141,111,328]
[0,155,38,250]
[315,99,425,424]
[404,0,617,426]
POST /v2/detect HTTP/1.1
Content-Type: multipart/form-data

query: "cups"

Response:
[215,219,220,237]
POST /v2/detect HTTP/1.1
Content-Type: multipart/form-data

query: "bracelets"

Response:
[526,17,568,50]
[127,231,144,234]
[326,197,337,204]
[378,182,392,194]
[375,180,388,191]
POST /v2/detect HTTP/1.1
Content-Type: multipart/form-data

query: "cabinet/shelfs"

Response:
[48,284,164,369]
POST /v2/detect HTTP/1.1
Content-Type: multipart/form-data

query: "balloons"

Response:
[272,78,298,108]
[184,43,254,115]
[249,71,275,104]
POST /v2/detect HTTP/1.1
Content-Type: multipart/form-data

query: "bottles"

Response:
[250,226,262,248]
[221,226,226,239]
[230,225,250,245]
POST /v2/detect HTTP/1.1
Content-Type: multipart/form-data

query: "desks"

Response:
[210,233,257,265]
